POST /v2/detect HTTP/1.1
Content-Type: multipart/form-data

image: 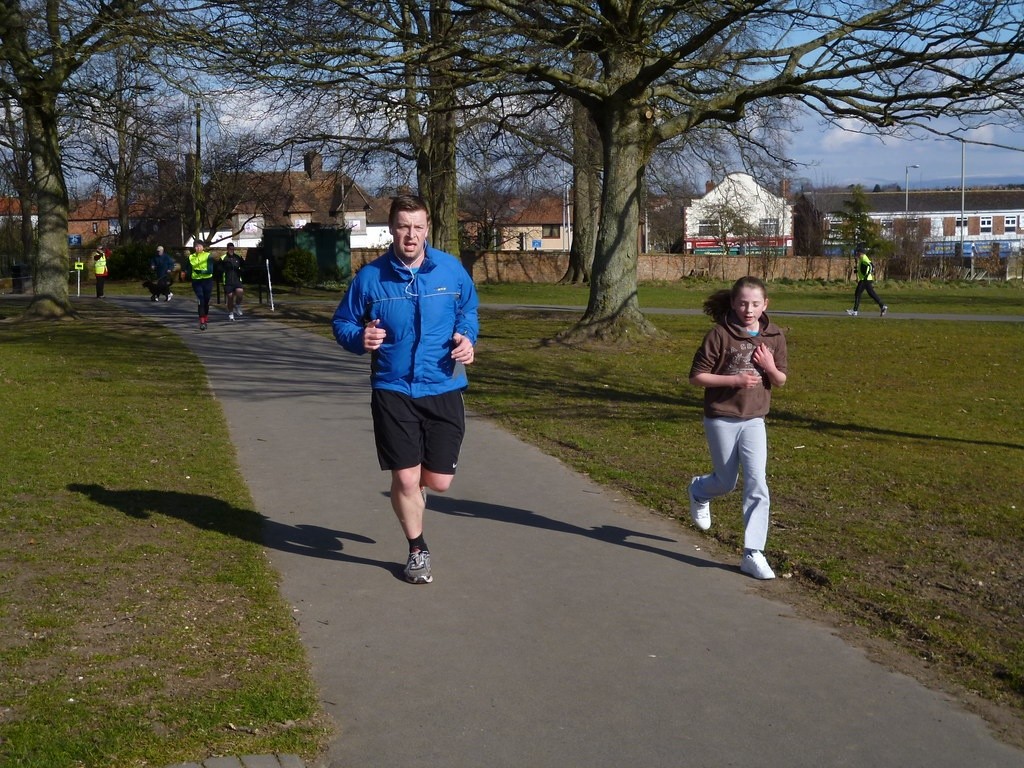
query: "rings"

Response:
[468,351,473,356]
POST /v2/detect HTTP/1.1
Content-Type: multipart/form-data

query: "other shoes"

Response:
[99,295,106,298]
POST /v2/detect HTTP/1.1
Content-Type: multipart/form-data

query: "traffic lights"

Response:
[516,232,526,251]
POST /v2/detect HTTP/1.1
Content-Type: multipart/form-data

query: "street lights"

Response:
[905,164,920,217]
[934,138,965,268]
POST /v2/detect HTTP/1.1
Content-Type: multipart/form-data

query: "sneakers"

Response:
[848,309,857,315]
[236,308,243,316]
[741,551,775,578]
[880,306,888,316]
[403,544,433,583]
[167,292,173,301]
[229,315,235,322]
[200,324,207,331]
[686,486,711,530]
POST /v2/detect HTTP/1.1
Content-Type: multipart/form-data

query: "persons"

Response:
[188,240,247,330]
[330,194,481,585]
[151,246,175,301]
[92,245,109,298]
[688,276,788,579]
[846,248,888,318]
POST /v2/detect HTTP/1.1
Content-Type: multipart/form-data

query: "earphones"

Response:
[389,227,393,233]
[425,228,428,238]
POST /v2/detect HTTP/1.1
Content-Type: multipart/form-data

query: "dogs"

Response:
[142,277,174,302]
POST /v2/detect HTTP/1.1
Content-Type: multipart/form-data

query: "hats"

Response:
[857,248,865,254]
[97,245,104,250]
[157,246,164,251]
